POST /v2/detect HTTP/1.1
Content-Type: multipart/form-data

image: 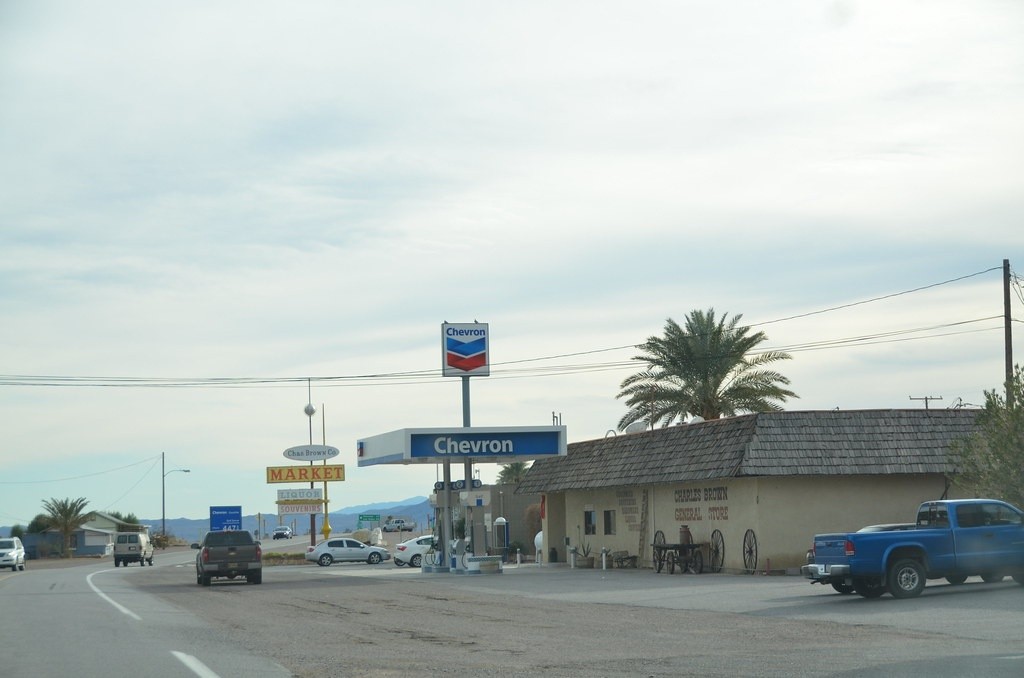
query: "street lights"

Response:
[162,469,190,550]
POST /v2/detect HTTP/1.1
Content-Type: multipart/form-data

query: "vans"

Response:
[113,531,155,567]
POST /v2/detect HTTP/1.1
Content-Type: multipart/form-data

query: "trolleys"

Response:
[649,543,705,576]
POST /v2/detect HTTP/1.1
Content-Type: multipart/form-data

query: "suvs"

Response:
[0,536,26,572]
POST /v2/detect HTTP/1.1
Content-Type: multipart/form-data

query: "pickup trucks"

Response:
[382,518,415,532]
[800,499,1024,600]
[190,531,263,587]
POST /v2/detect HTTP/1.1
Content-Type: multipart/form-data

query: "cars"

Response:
[805,523,917,593]
[394,534,434,568]
[272,525,293,540]
[305,538,391,567]
